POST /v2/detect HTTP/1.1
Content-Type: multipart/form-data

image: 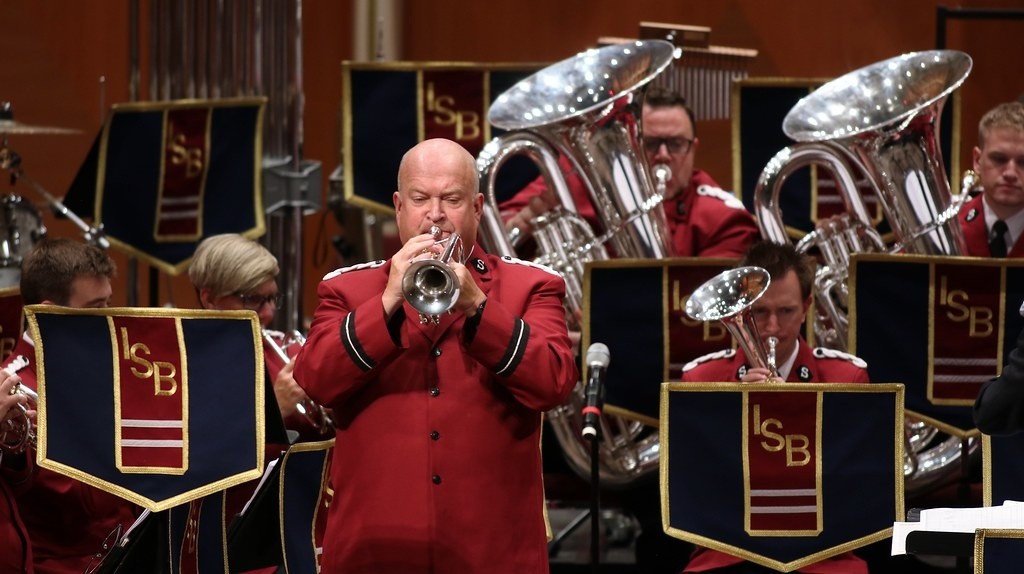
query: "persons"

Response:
[936,102,1024,497]
[187,233,334,573]
[293,138,579,574]
[7,236,136,574]
[0,368,36,574]
[680,240,869,574]
[502,153,590,249]
[582,86,762,259]
[972,302,1024,437]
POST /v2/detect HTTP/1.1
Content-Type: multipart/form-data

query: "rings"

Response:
[409,258,412,264]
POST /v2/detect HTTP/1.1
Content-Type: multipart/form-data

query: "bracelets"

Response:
[467,301,485,327]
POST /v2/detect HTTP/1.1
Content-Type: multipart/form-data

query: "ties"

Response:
[988,220,1009,259]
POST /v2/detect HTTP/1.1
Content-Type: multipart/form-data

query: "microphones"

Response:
[583,342,610,439]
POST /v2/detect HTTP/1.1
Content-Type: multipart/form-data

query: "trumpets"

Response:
[402,226,465,313]
[682,267,784,382]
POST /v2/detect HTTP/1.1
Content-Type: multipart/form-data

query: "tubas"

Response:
[476,39,679,484]
[755,47,973,350]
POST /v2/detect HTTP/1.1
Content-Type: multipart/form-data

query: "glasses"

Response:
[644,137,694,154]
[232,292,284,313]
